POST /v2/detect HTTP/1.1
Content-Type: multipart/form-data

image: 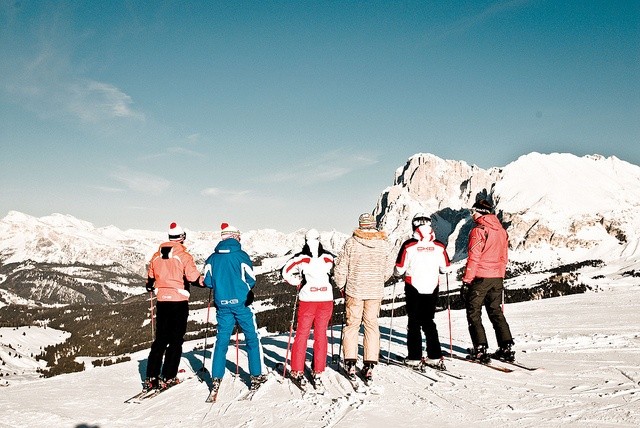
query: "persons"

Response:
[392,211,452,368]
[279,226,337,381]
[332,212,395,380]
[140,221,205,392]
[460,198,516,366]
[202,222,267,392]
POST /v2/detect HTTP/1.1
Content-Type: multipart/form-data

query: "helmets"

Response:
[412,212,432,233]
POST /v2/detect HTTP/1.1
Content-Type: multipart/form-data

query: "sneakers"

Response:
[289,371,304,379]
[158,377,180,390]
[145,377,162,389]
[311,371,322,378]
[493,342,512,356]
[360,363,374,376]
[343,361,357,375]
[212,378,223,385]
[404,360,422,366]
[251,374,268,383]
[471,344,487,358]
[424,357,442,366]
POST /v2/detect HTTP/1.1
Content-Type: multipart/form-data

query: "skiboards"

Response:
[125,369,198,404]
[274,362,326,395]
[380,354,463,382]
[204,372,271,403]
[332,354,373,392]
[441,348,538,372]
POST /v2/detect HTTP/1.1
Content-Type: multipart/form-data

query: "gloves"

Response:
[460,282,471,304]
[146,283,155,292]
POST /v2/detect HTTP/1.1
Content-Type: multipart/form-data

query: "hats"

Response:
[359,213,377,229]
[169,222,184,243]
[305,228,320,240]
[472,200,492,215]
[221,223,241,241]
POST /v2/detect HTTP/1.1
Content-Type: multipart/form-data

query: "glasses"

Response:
[169,232,186,240]
[468,208,490,217]
